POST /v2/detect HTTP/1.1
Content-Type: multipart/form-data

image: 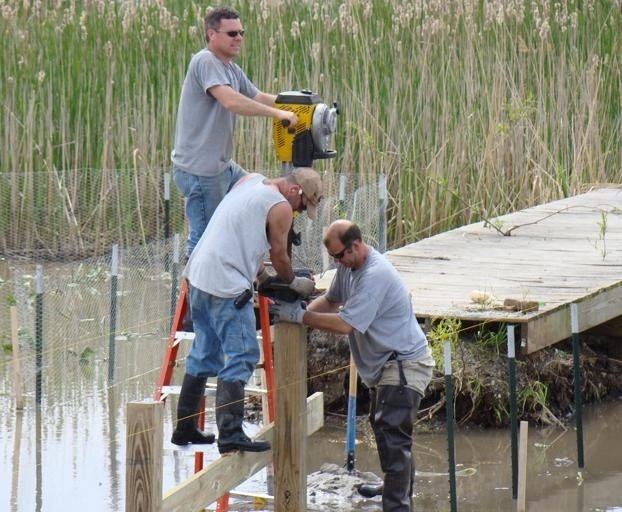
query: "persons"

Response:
[268,220,435,511]
[170,168,325,453]
[172,4,301,330]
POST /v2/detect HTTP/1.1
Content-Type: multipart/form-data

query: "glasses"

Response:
[298,188,306,211]
[335,247,346,258]
[218,30,244,37]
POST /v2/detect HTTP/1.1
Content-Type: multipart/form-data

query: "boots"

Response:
[172,373,214,445]
[216,379,270,451]
[359,387,416,511]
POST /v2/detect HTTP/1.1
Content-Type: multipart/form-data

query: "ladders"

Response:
[153,276,274,512]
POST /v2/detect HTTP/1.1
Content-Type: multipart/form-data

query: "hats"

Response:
[291,167,323,220]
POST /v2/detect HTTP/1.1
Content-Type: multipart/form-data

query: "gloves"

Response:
[289,276,315,298]
[257,267,273,291]
[268,298,310,327]
